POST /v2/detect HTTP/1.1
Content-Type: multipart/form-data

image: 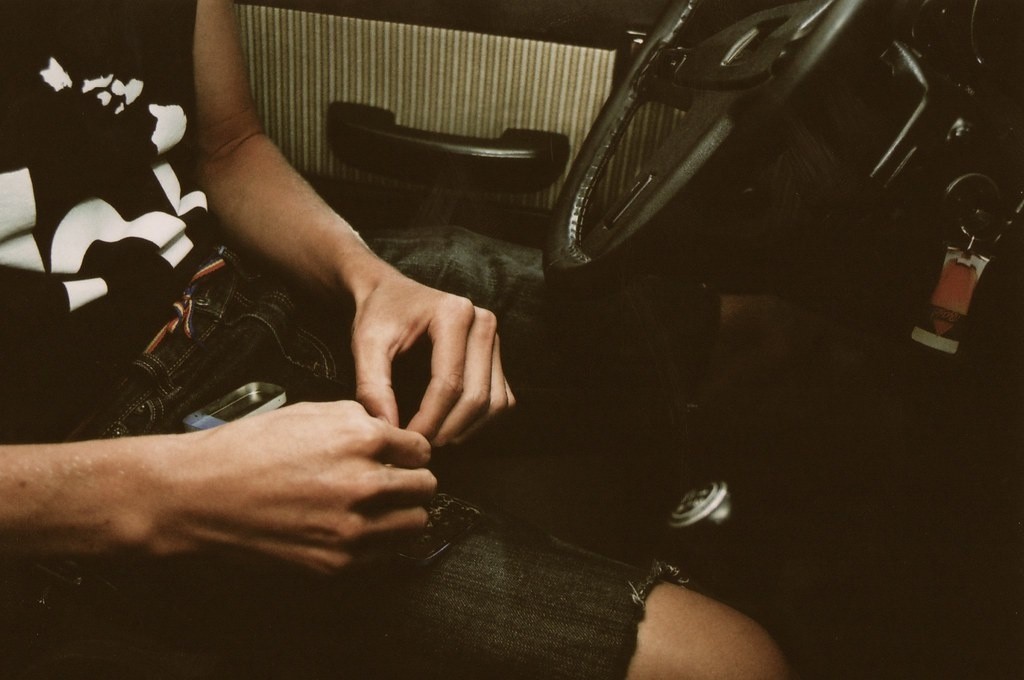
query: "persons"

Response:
[1,2,861,679]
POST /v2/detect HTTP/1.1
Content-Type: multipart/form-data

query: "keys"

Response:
[945,202,1003,247]
